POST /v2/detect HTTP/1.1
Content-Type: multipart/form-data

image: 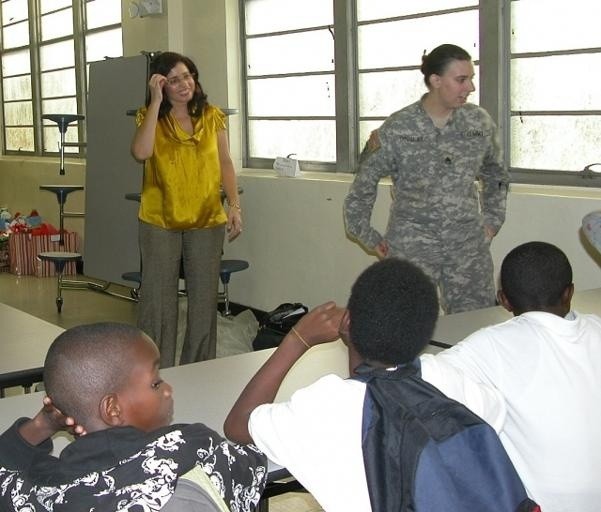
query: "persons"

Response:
[131,54,245,369]
[418,241,601,512]
[344,44,512,319]
[220,258,543,512]
[0,321,270,512]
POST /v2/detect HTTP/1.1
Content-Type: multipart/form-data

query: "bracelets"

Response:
[290,325,311,351]
[227,200,240,212]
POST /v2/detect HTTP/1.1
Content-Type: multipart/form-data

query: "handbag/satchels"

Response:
[252,303,308,351]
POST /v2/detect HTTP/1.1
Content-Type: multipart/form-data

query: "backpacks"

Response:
[344,356,541,512]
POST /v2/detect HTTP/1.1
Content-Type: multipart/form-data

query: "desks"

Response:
[0,296,79,399]
[427,290,599,350]
[0,333,451,511]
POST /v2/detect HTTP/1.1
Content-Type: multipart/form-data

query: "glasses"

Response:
[162,72,195,85]
